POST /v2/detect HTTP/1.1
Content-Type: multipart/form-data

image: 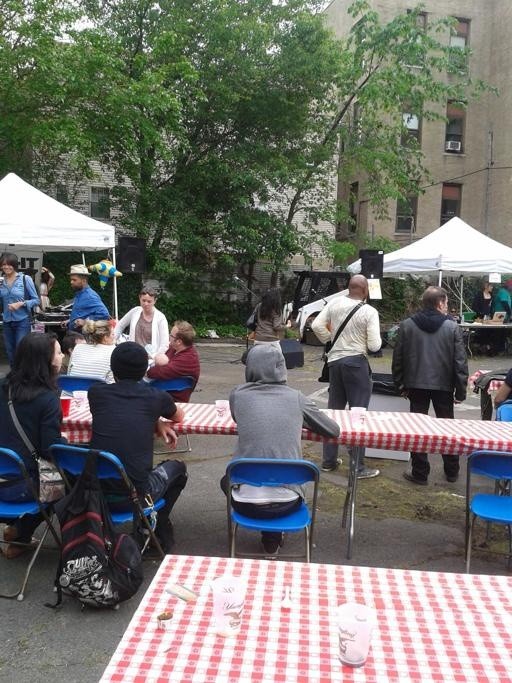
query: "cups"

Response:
[73,391,88,410]
[211,577,245,635]
[215,400,229,422]
[351,406,365,429]
[336,604,371,666]
[59,396,71,418]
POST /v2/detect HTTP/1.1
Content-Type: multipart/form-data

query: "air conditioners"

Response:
[446,141,461,150]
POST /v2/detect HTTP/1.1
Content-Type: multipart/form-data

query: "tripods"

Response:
[230,285,262,364]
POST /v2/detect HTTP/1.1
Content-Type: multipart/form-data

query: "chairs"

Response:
[52,444,167,611]
[0,448,61,601]
[226,460,318,564]
[463,452,512,574]
[148,375,196,455]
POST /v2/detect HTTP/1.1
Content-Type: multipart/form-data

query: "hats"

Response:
[66,265,93,275]
[503,279,512,287]
[40,272,49,282]
[112,341,148,377]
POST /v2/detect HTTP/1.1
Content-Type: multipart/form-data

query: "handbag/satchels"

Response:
[23,274,46,334]
[472,369,511,394]
[247,307,258,330]
[47,284,54,304]
[8,385,65,503]
[318,301,365,383]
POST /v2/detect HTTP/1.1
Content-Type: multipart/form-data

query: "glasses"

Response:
[168,332,180,341]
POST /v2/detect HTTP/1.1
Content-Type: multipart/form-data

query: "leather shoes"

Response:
[1,520,35,543]
[447,472,458,482]
[404,469,428,486]
[0,537,41,558]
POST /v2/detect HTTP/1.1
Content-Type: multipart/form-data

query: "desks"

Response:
[470,370,511,420]
[462,322,512,358]
[58,397,512,558]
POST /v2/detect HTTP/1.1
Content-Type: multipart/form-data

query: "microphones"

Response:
[311,288,321,299]
[231,272,246,285]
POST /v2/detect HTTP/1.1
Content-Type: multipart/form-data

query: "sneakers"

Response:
[261,533,281,559]
[356,468,380,479]
[137,533,175,559]
[270,531,285,548]
[321,457,342,471]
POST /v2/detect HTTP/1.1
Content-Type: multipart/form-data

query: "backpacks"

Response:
[50,447,143,607]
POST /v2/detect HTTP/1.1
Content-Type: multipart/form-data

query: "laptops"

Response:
[486,312,507,322]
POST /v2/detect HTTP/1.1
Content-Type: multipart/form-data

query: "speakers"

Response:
[281,339,304,368]
[359,249,384,280]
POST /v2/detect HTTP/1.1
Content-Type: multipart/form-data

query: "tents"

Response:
[0,171,118,325]
[344,214,512,323]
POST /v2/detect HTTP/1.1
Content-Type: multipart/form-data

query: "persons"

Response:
[60,264,111,336]
[145,320,201,404]
[253,292,292,354]
[0,332,71,559]
[472,281,494,355]
[220,344,340,560]
[40,267,56,311]
[311,274,382,480]
[0,253,40,370]
[114,286,169,385]
[391,286,469,485]
[86,341,188,558]
[494,370,512,410]
[68,320,116,384]
[494,279,512,355]
[55,332,86,403]
[450,308,462,324]
